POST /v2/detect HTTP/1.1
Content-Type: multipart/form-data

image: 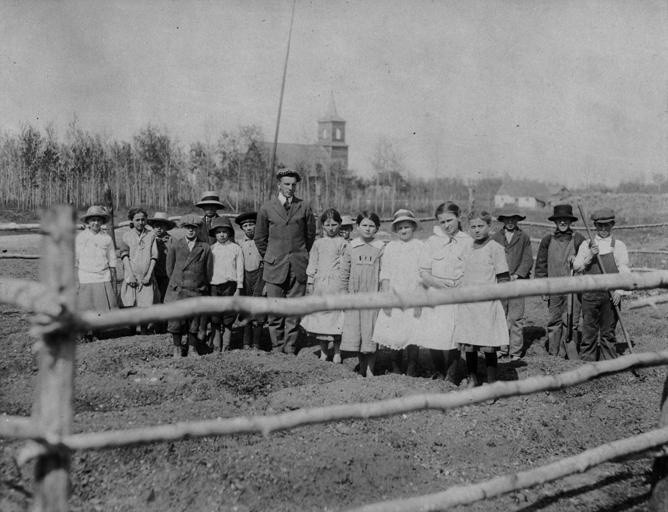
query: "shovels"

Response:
[564,260,579,362]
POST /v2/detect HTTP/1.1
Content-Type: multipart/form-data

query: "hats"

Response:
[548,203,580,222]
[236,209,259,226]
[589,206,618,223]
[193,190,229,207]
[389,209,423,230]
[148,210,176,230]
[207,216,234,237]
[497,207,526,222]
[179,214,203,234]
[79,206,111,221]
[276,169,302,181]
